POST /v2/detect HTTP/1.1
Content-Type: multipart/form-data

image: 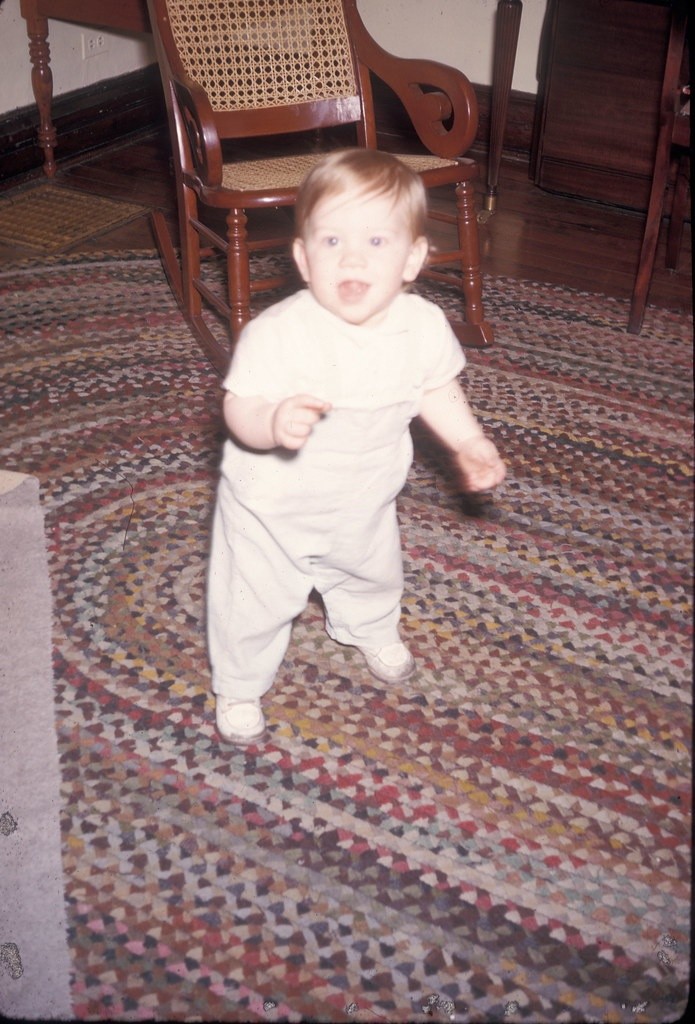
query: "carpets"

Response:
[0,245,695,1024]
[0,179,146,253]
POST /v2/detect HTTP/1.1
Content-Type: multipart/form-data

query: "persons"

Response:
[207,150,507,747]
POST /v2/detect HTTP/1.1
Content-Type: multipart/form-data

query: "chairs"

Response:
[146,0,494,379]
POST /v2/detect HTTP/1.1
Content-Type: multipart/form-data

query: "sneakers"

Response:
[216,693,267,746]
[353,631,416,684]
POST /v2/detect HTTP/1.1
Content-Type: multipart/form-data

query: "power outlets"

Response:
[80,31,110,57]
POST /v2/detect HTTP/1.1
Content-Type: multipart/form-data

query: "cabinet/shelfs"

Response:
[535,1,694,220]
